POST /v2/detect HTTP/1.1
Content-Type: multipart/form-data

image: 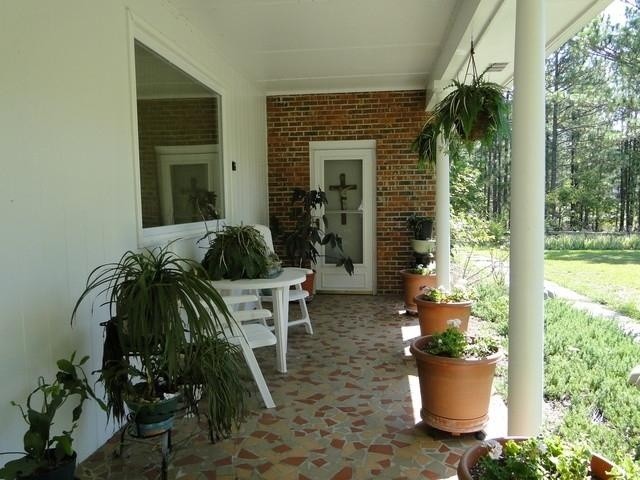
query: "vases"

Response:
[456,436,629,480]
[413,294,476,335]
[409,334,502,433]
[400,269,437,309]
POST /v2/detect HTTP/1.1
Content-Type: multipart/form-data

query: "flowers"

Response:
[428,317,469,357]
[423,279,479,302]
[400,261,437,275]
[477,437,624,480]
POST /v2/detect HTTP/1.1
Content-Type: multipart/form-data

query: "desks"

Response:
[207,270,307,374]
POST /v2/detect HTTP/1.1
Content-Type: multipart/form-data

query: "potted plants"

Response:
[67,238,243,380]
[410,62,510,169]
[0,344,109,480]
[408,215,436,254]
[122,340,247,443]
[282,187,354,303]
[197,223,282,280]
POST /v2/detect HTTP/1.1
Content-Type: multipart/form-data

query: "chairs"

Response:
[239,225,313,339]
[177,295,279,409]
